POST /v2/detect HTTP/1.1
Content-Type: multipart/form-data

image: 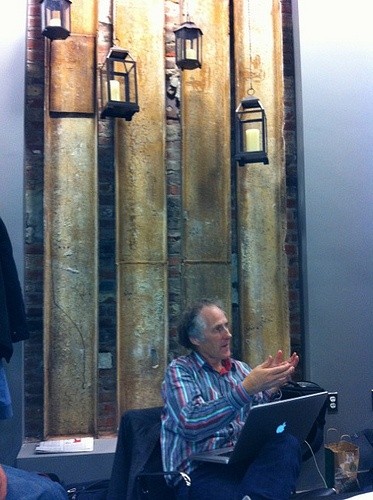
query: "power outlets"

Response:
[325,392,338,415]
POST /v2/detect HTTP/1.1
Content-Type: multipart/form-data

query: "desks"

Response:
[14,436,120,500]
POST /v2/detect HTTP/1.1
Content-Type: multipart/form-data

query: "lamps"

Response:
[172,0,206,74]
[96,0,141,123]
[37,0,73,42]
[224,0,272,170]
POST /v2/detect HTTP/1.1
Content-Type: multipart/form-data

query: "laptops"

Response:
[189,390,328,464]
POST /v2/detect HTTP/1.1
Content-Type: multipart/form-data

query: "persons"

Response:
[0,220,69,500]
[162,298,298,500]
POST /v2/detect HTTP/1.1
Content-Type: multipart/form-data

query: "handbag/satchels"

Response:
[326,434,361,494]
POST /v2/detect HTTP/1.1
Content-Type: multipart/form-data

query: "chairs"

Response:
[117,405,193,500]
